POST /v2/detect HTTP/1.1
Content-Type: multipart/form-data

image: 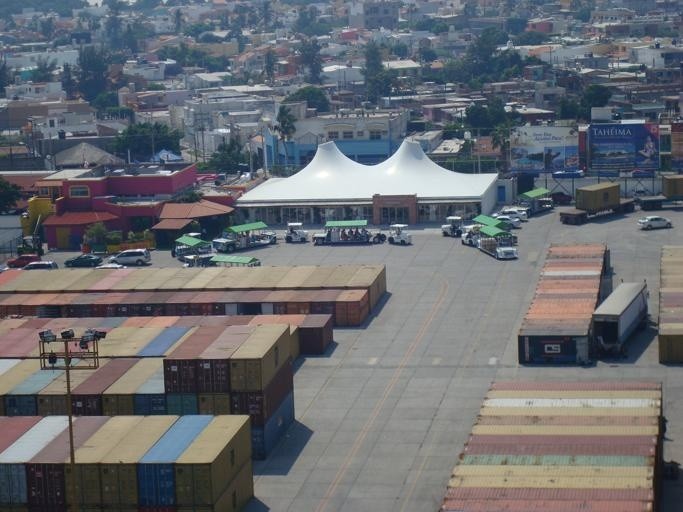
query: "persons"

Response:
[341,227,367,241]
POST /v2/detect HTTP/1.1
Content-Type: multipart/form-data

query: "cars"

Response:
[548,192,572,204]
[6,234,58,270]
[87,237,120,254]
[64,254,102,267]
[637,216,671,230]
[494,209,529,226]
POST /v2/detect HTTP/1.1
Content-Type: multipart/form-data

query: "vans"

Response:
[107,249,150,266]
